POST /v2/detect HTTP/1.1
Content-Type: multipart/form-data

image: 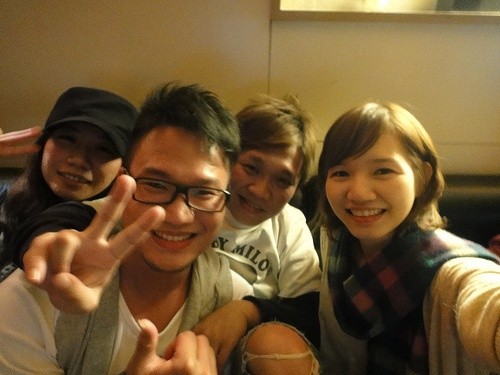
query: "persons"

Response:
[0,86,140,286]
[305,101,500,375]
[0,82,322,375]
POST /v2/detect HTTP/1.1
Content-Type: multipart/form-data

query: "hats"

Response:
[42,86,139,157]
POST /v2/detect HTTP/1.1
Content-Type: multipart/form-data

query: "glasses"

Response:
[127,170,233,213]
